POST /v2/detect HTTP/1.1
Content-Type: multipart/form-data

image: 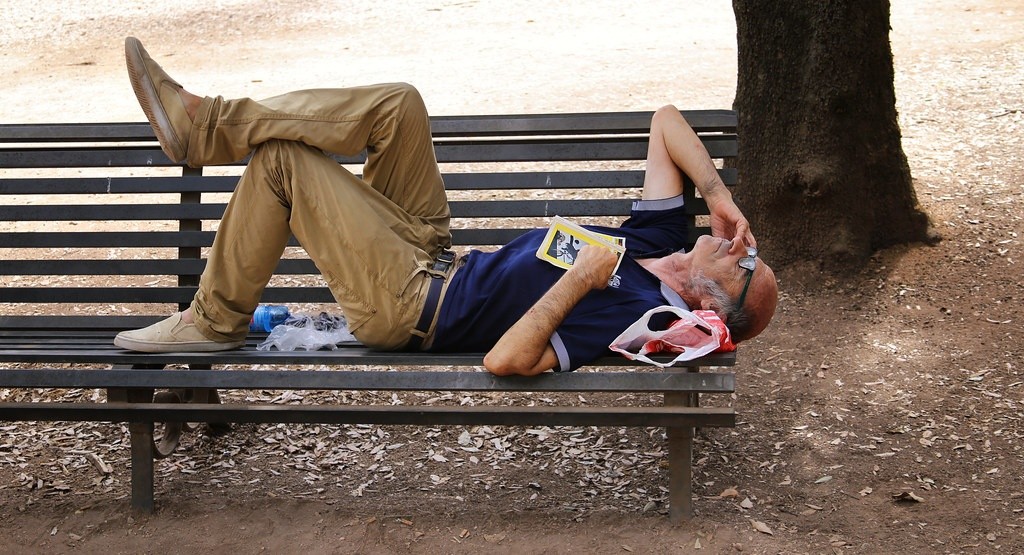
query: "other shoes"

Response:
[125,37,193,163]
[114,313,247,353]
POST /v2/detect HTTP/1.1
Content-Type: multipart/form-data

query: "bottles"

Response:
[249,306,291,332]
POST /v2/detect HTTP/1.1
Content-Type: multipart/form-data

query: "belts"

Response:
[406,249,456,351]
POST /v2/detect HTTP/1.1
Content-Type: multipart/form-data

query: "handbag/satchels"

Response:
[608,305,739,367]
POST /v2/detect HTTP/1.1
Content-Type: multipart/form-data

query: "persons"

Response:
[113,37,778,377]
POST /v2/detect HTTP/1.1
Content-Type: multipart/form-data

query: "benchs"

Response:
[0,110,742,513]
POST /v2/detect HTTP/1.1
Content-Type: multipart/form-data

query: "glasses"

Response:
[726,246,758,328]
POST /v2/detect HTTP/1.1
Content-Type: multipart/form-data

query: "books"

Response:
[535,214,626,280]
